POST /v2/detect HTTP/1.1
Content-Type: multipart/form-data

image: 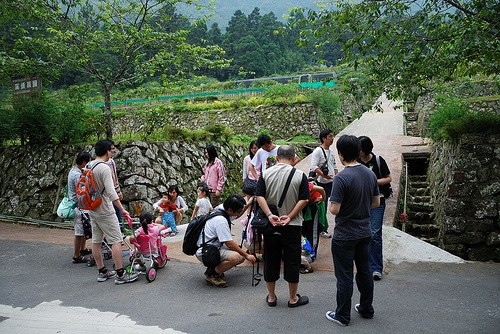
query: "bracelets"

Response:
[267,213,271,218]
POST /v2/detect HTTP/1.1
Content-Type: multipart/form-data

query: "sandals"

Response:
[80,248,93,255]
[73,255,88,263]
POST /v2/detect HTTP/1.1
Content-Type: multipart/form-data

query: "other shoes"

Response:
[325,311,349,326]
[354,304,374,318]
[135,265,146,271]
[373,271,381,281]
[300,267,313,273]
[320,232,331,238]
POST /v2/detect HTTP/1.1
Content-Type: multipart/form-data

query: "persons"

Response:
[255,144,309,307]
[303,182,328,255]
[67,152,92,263]
[243,135,300,186]
[202,144,225,209]
[358,135,392,280]
[242,180,264,254]
[104,140,124,220]
[130,212,166,258]
[153,185,188,236]
[300,234,316,274]
[86,140,138,284]
[325,135,381,326]
[311,130,338,238]
[191,185,213,220]
[195,195,256,286]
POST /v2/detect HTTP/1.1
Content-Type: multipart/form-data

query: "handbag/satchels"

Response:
[242,176,256,196]
[57,198,75,218]
[381,183,393,199]
[201,245,220,266]
[251,205,282,234]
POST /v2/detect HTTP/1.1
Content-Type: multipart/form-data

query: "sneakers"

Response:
[204,270,227,289]
[114,271,139,284]
[97,270,116,282]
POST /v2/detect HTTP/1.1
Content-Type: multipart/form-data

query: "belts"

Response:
[209,189,215,192]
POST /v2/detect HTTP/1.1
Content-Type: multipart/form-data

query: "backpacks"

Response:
[181,208,232,255]
[75,162,114,210]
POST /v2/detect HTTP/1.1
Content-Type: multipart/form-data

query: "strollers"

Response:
[77,208,133,267]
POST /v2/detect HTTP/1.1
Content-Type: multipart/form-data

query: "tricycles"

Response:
[122,213,171,282]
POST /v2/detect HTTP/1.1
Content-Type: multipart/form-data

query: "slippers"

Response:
[266,294,278,307]
[287,294,308,308]
